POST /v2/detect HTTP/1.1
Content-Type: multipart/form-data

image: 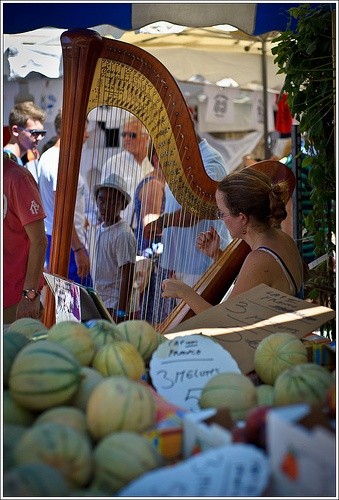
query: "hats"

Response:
[93,173,132,204]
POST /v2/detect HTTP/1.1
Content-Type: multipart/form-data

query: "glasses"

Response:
[121,131,148,139]
[18,126,47,137]
[214,208,237,220]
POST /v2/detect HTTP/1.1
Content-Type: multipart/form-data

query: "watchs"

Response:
[20,289,40,301]
[115,309,127,318]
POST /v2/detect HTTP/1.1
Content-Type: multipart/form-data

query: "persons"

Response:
[36,108,92,289]
[2,101,46,187]
[88,174,136,328]
[161,167,306,316]
[139,104,232,320]
[101,116,158,243]
[3,151,49,328]
[133,145,172,323]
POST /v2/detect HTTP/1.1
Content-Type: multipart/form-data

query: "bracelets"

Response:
[73,246,85,254]
